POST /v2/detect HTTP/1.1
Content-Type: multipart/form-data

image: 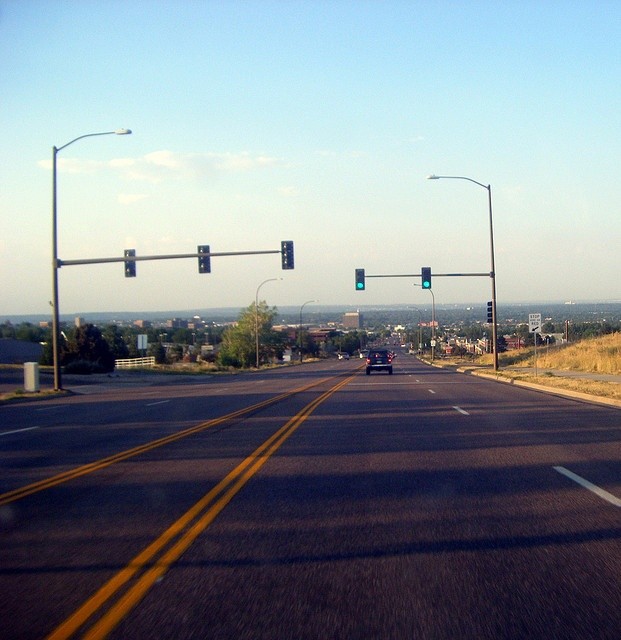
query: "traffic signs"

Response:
[528,313,540,333]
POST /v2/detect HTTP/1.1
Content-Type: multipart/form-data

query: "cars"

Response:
[338,352,349,360]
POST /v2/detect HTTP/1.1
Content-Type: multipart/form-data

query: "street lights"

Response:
[51,128,134,390]
[255,277,284,367]
[406,305,423,356]
[411,283,435,361]
[426,174,497,371]
[300,299,320,363]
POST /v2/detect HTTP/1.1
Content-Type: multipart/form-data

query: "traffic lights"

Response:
[421,267,431,289]
[354,269,364,290]
[487,302,492,323]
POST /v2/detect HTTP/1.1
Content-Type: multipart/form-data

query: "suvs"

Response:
[360,350,369,359]
[366,348,392,375]
